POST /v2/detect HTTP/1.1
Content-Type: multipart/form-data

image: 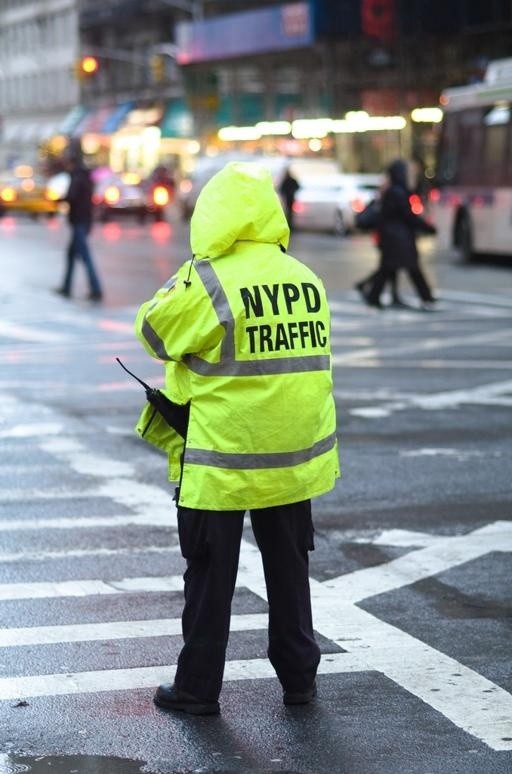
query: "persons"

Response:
[48,141,103,304]
[133,159,343,715]
[352,167,413,313]
[277,165,301,236]
[361,158,447,310]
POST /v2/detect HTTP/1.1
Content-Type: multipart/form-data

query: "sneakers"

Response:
[357,280,449,312]
[154,682,220,715]
[283,680,317,706]
[52,287,102,302]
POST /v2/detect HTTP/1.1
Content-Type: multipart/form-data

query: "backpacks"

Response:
[353,200,383,231]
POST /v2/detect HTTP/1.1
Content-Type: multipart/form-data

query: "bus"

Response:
[429,57,512,261]
